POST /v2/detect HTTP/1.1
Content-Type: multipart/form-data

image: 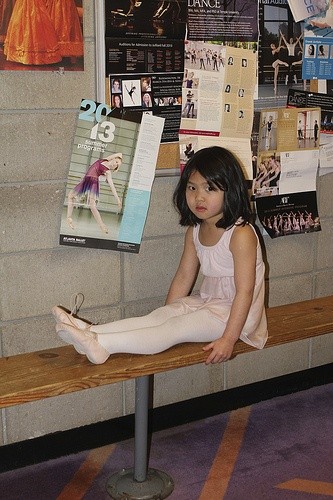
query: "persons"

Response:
[64,147,121,235]
[111,78,181,111]
[184,30,325,197]
[50,143,272,365]
[262,207,322,237]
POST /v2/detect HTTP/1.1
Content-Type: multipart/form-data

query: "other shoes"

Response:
[52,306,90,355]
[56,322,109,366]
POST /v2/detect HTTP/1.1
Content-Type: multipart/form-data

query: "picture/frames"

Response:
[93,0,333,180]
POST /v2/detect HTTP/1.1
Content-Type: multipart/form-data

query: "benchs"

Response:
[1,296,332,500]
